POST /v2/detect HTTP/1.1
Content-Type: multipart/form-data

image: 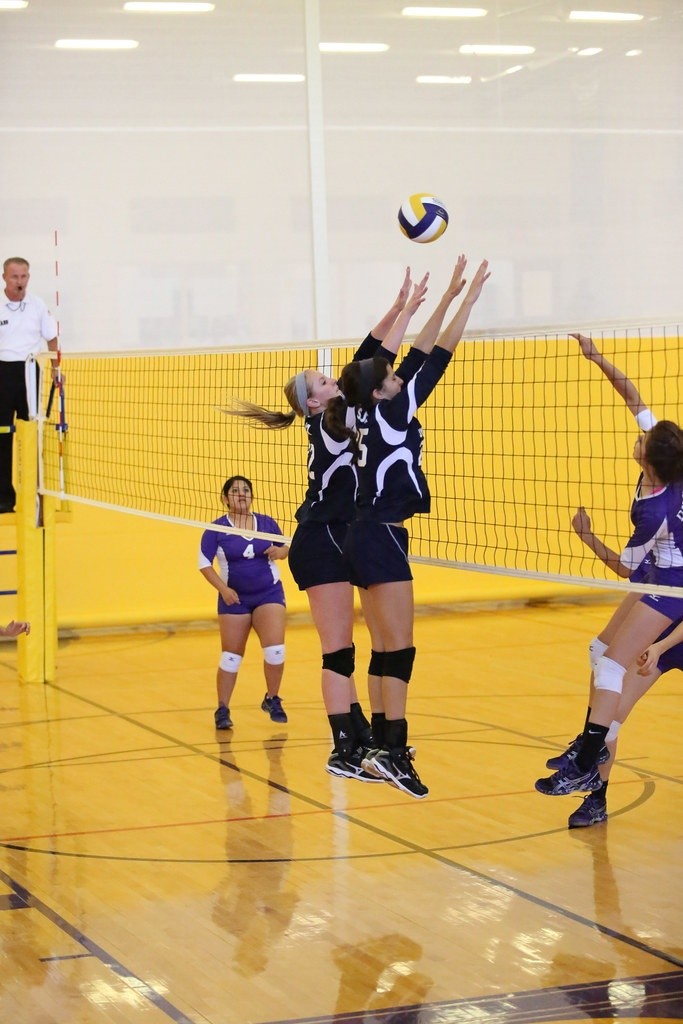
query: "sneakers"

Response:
[361,749,429,798]
[360,732,417,761]
[214,702,233,728]
[546,734,610,770]
[325,744,386,783]
[261,692,287,723]
[535,759,603,796]
[569,794,607,826]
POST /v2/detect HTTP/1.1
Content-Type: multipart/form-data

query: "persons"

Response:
[326,255,494,800]
[195,476,294,731]
[219,263,431,783]
[211,733,300,979]
[540,821,683,1023]
[0,619,31,638]
[0,257,67,515]
[534,331,682,828]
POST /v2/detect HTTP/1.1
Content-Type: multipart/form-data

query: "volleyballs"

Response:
[397,193,449,244]
